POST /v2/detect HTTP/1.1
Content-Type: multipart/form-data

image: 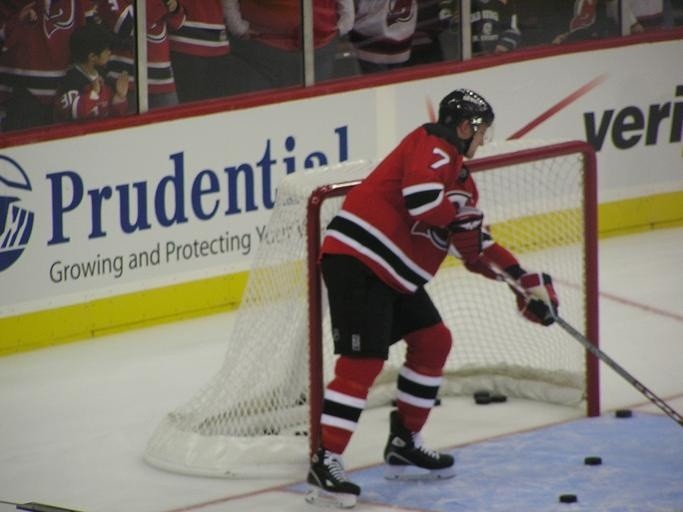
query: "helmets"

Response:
[438,89,495,159]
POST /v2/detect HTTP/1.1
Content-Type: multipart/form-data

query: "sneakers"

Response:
[384,412,454,470]
[308,450,360,496]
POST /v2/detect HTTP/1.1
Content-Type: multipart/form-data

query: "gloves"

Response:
[515,274,559,325]
[452,207,483,261]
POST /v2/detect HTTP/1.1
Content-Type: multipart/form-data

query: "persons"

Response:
[305,88,559,496]
[1,1,682,134]
